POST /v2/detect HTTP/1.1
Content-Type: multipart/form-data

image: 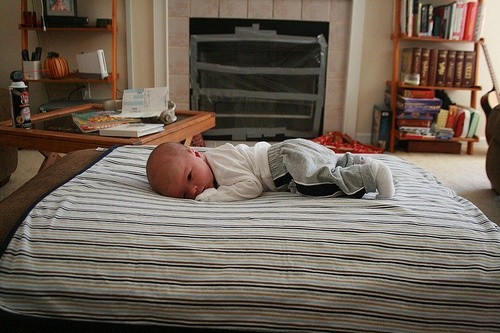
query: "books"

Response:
[401,48,476,88]
[99,124,165,137]
[400,0,478,41]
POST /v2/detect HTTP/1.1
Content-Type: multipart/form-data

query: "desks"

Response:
[0,103,216,153]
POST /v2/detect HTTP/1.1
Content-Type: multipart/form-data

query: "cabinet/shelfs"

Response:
[386,0,485,155]
[18,0,119,101]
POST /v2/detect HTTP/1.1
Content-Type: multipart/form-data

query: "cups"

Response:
[23,61,41,80]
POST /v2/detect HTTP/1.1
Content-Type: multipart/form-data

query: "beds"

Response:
[0,144,500,333]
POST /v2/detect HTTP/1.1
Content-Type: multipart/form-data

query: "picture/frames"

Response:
[43,0,78,16]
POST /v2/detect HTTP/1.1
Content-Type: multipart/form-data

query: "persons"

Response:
[146,139,394,202]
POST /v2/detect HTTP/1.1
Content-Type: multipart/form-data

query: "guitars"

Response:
[479,38,500,147]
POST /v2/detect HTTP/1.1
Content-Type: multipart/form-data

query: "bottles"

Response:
[8,71,32,130]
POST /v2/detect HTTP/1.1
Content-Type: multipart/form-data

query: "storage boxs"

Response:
[371,90,449,151]
[23,61,43,80]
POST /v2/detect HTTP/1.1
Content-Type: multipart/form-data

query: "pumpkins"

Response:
[44,51,69,79]
